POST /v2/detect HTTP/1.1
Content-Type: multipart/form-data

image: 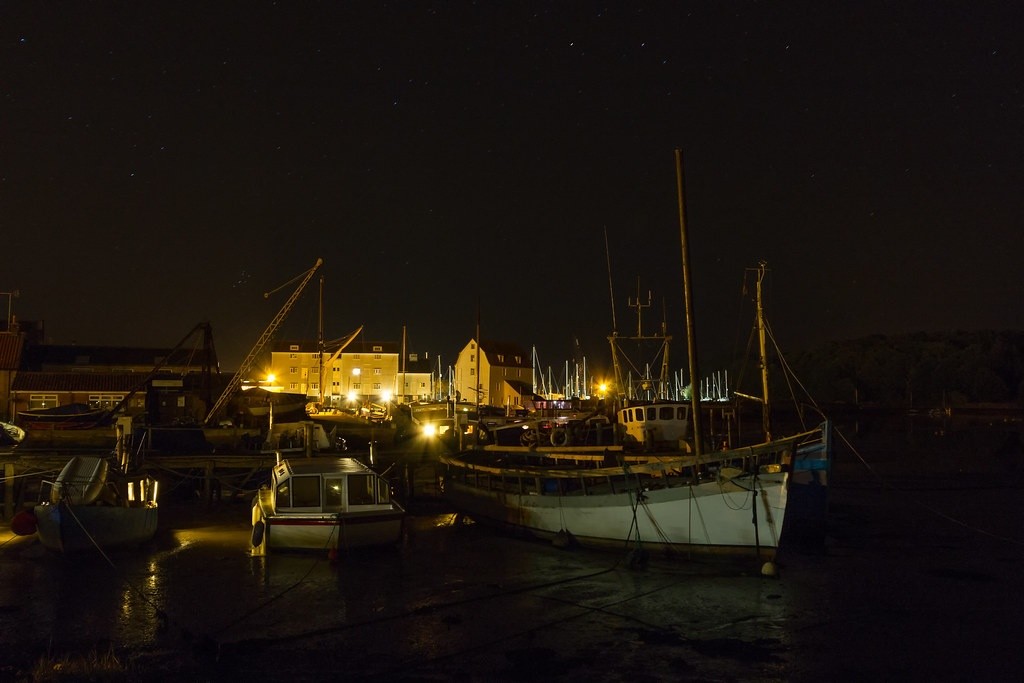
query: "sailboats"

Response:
[303,148,832,563]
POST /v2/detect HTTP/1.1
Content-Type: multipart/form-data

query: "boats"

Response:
[256,420,331,453]
[15,402,108,431]
[9,454,162,553]
[249,439,407,556]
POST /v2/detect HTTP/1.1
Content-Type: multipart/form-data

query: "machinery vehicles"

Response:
[177,254,325,428]
